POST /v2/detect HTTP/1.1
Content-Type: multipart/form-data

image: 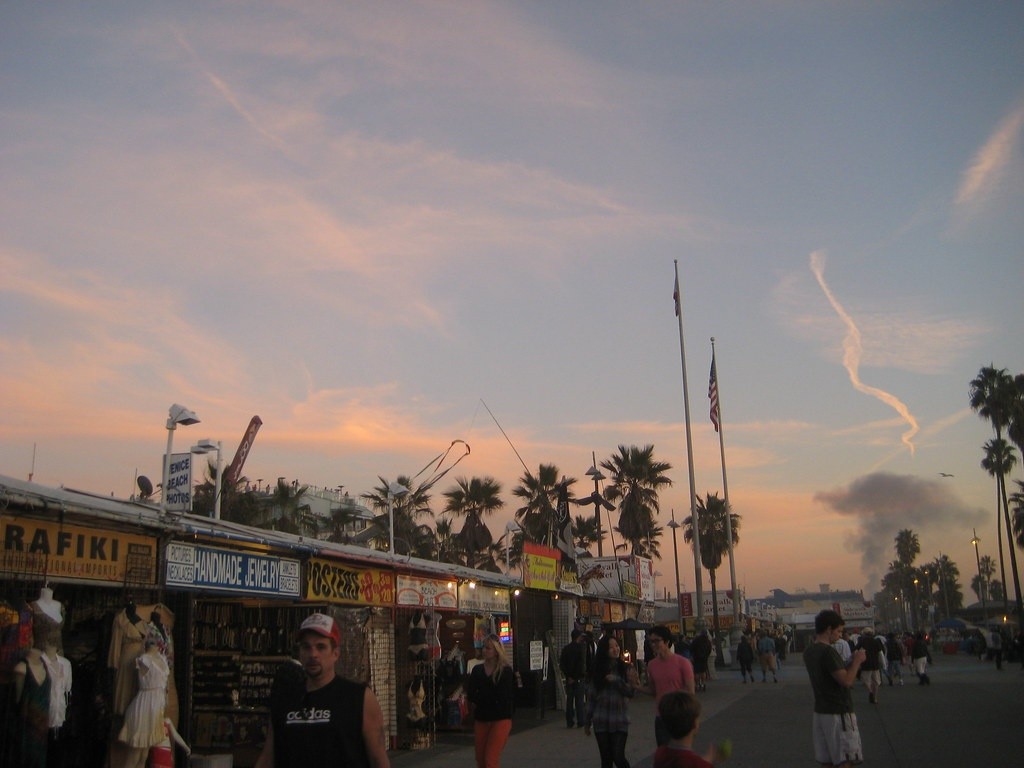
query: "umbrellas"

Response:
[933,615,1018,633]
[608,616,652,649]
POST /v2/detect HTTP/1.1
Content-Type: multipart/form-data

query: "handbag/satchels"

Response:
[877,640,887,671]
[901,655,906,665]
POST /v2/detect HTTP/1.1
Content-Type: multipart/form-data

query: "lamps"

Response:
[159,403,201,517]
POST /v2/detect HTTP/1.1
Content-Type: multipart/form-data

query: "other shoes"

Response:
[888,679,893,686]
[899,679,904,688]
[869,693,879,706]
[920,677,930,685]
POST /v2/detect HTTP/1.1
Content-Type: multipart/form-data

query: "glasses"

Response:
[648,639,665,644]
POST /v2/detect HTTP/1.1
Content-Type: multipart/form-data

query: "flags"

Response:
[673,269,680,318]
[554,482,576,566]
[708,354,719,433]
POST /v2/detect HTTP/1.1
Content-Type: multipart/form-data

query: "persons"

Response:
[12,589,191,768]
[559,629,791,768]
[253,613,394,768]
[405,608,443,733]
[833,625,1024,706]
[803,609,866,768]
[467,634,518,768]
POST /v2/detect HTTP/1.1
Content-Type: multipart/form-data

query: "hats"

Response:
[296,612,340,645]
[861,627,875,639]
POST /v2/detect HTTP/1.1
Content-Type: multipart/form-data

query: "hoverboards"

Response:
[694,673,706,691]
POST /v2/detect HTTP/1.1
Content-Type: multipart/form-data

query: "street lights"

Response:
[161,402,201,510]
[189,438,225,520]
[895,588,906,631]
[924,563,935,625]
[970,528,988,630]
[667,508,682,607]
[938,550,951,619]
[504,520,522,575]
[387,481,410,553]
[913,574,922,630]
[585,449,606,557]
